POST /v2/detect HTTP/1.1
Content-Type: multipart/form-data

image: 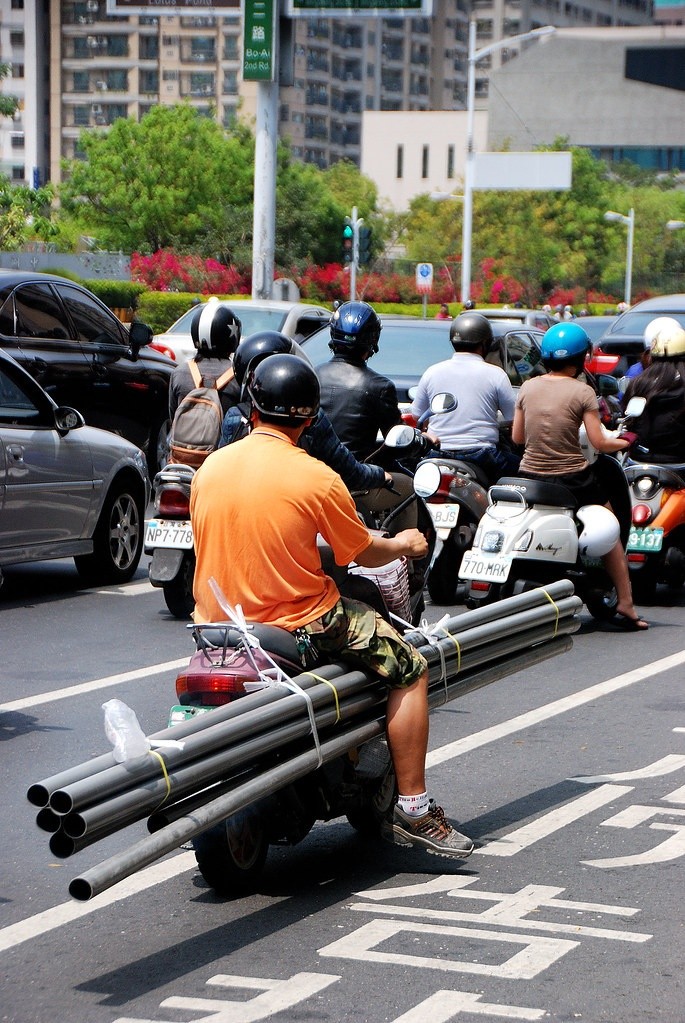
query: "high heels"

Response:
[612,608,648,632]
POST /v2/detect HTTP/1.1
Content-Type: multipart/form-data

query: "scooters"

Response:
[144,384,523,636]
[167,459,443,893]
[454,375,685,620]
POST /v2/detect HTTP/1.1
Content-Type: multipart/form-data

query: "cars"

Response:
[0,349,149,585]
[140,299,336,369]
[563,293,685,386]
[293,308,622,462]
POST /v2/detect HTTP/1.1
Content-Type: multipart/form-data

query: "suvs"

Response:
[0,267,178,496]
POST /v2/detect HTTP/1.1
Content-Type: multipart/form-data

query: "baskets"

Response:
[353,554,413,632]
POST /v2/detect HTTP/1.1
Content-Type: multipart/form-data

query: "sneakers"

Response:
[391,802,473,858]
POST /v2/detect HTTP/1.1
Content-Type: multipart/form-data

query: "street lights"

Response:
[460,26,555,309]
[604,209,635,309]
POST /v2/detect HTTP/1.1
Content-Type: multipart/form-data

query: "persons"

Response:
[621,317,685,484]
[511,323,652,633]
[190,353,475,861]
[313,299,441,573]
[167,302,244,469]
[220,330,392,529]
[434,299,627,320]
[406,313,519,484]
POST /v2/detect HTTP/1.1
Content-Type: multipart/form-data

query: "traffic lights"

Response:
[359,227,372,262]
[343,224,353,262]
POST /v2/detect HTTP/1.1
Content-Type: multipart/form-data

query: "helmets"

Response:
[449,313,493,346]
[540,322,589,362]
[241,352,320,427]
[328,301,381,358]
[233,333,297,394]
[189,304,240,355]
[645,316,681,349]
[651,327,685,357]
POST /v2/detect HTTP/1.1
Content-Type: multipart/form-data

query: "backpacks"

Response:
[167,358,238,469]
